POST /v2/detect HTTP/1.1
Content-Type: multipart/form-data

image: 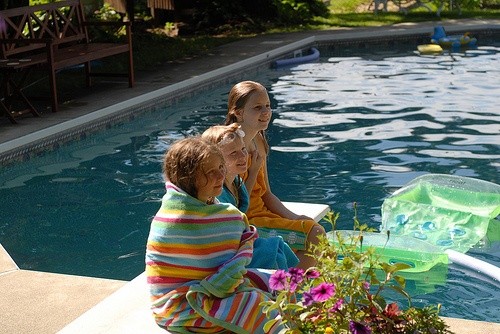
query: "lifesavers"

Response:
[275,46,320,66]
[446,249,500,280]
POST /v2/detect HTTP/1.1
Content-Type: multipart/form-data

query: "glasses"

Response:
[217,128,245,144]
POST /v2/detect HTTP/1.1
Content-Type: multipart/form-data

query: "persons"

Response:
[224,81,336,268]
[203,122,301,273]
[146,139,301,334]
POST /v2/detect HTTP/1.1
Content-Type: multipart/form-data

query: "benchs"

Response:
[53,200,331,334]
[0,0,135,112]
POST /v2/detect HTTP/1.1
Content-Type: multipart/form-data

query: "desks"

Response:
[0,58,49,124]
